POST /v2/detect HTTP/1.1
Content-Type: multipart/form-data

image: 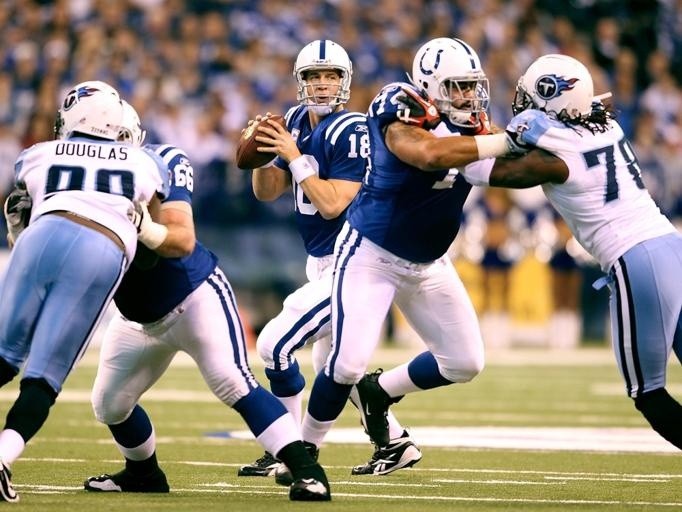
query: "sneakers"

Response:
[0,460,20,504]
[83,470,170,492]
[352,367,405,448]
[240,442,420,498]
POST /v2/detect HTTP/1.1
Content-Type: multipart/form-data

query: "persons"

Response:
[238,40,424,477]
[0,80,165,502]
[394,54,682,453]
[1,1,682,349]
[273,35,572,487]
[1,100,334,501]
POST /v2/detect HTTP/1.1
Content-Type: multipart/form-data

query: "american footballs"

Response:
[236,115,286,170]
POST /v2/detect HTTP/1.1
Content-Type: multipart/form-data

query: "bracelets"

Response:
[288,154,316,184]
[474,134,509,160]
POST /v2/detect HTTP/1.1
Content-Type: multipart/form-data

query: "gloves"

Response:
[126,191,168,250]
[396,83,442,130]
[2,188,32,241]
[501,107,548,156]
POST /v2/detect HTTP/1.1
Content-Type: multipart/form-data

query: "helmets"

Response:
[292,37,354,118]
[117,97,146,147]
[54,79,124,141]
[412,36,484,121]
[517,53,595,119]
